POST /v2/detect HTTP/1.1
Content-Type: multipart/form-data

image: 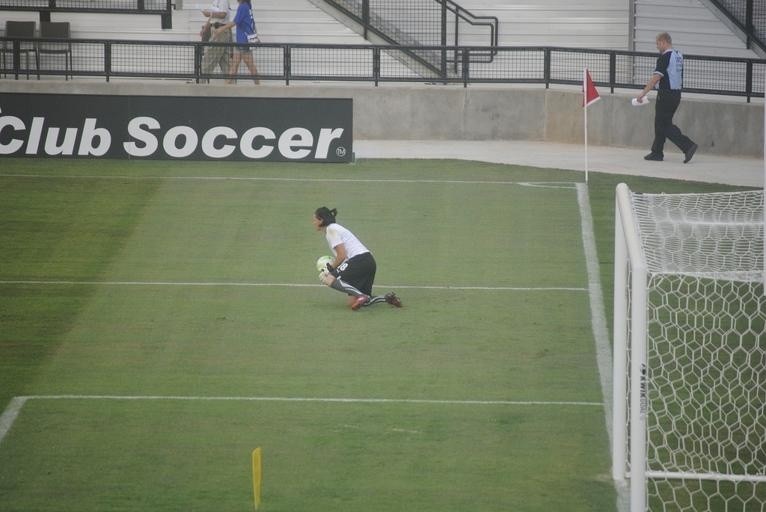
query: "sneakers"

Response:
[644,152,664,161]
[352,293,369,310]
[384,291,402,308]
[684,143,697,163]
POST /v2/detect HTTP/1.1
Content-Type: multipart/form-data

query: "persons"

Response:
[188,0,231,85]
[310,205,404,310]
[213,1,263,85]
[634,34,699,162]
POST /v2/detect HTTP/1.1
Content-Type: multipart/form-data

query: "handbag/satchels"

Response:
[247,34,261,51]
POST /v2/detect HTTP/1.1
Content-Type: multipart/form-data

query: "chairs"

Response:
[24,21,74,83]
[0,20,38,82]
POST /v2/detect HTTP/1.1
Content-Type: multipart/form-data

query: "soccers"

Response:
[318,256,334,272]
